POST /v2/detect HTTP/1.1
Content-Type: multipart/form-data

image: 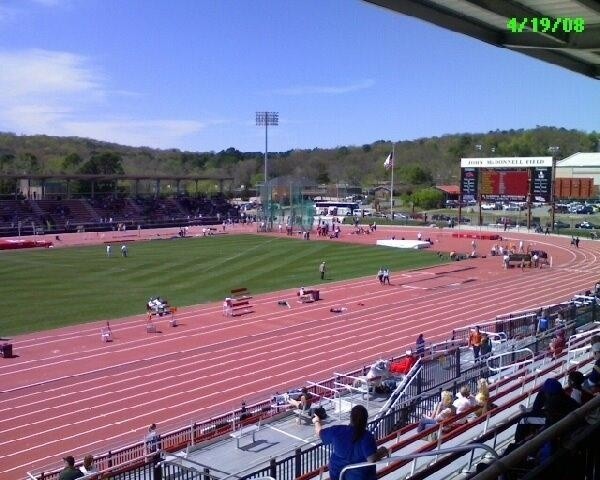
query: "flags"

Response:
[383,151,393,172]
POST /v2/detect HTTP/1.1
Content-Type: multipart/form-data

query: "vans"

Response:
[353,209,372,217]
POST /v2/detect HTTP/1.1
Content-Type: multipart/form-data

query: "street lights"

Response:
[475,145,495,157]
[256,112,279,183]
[547,146,562,230]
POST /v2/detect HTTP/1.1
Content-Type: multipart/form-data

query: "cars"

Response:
[481,201,543,211]
[555,201,600,215]
[373,211,409,219]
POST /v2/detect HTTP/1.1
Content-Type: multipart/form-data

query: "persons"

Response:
[449,218,454,228]
[149,296,167,314]
[378,268,390,284]
[504,221,579,247]
[285,307,600,480]
[121,246,128,256]
[417,232,421,240]
[106,244,111,254]
[148,424,160,452]
[2,194,68,228]
[90,190,376,241]
[300,287,304,296]
[58,455,100,480]
[438,240,538,270]
[319,262,326,280]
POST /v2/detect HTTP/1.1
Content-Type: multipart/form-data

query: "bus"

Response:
[315,201,360,216]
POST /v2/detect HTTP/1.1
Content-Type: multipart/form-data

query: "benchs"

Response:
[230,422,260,449]
